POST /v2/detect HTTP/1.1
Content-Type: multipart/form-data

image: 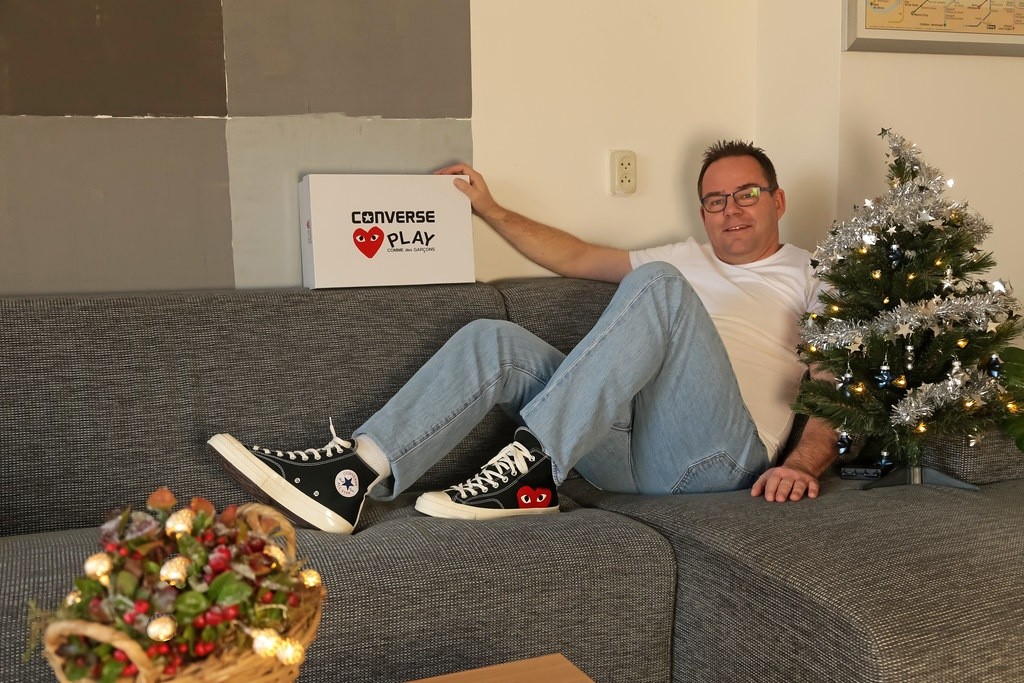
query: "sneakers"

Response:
[414,426,560,519]
[206,417,384,534]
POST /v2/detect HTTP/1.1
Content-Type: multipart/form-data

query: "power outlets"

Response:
[610,150,636,194]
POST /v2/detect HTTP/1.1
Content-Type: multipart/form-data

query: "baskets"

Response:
[42,498,324,683]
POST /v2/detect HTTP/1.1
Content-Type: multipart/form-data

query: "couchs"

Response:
[1,277,1024,682]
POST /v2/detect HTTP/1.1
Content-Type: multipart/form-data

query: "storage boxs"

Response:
[298,173,476,289]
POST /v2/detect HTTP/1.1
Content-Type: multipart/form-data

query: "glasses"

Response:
[701,185,775,214]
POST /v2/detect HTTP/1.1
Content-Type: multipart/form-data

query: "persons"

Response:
[207,139,845,535]
